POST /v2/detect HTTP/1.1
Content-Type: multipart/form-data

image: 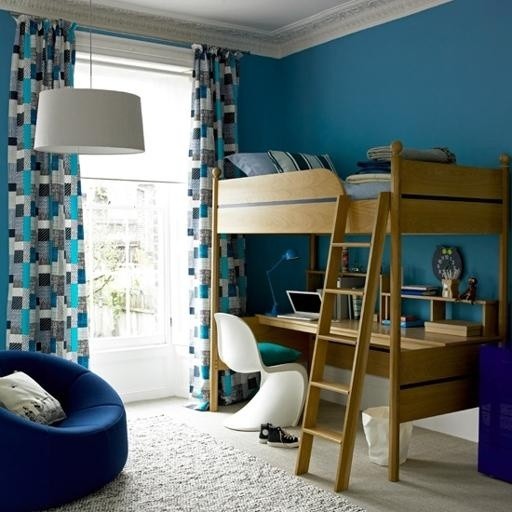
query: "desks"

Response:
[253,272,498,482]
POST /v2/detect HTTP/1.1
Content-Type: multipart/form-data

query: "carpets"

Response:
[51,412,367,512]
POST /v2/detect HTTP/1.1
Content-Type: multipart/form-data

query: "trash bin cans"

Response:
[362,406,412,465]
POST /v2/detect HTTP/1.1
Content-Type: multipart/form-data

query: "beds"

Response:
[209,140,510,492]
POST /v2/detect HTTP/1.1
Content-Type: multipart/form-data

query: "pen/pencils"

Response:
[441,269,460,279]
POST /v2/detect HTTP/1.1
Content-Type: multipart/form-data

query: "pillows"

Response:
[1,370,66,425]
[266,149,337,177]
[224,152,276,176]
[257,340,303,366]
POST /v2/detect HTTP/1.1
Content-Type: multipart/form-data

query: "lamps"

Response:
[34,2,146,155]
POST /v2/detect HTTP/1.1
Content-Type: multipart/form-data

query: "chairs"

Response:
[0,350,128,512]
[213,313,309,432]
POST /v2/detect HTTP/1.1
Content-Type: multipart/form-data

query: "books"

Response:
[317,273,482,337]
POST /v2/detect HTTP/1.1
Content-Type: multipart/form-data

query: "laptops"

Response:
[276,289,321,322]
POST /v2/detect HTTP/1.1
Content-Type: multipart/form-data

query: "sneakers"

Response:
[259,423,273,443]
[267,425,298,448]
